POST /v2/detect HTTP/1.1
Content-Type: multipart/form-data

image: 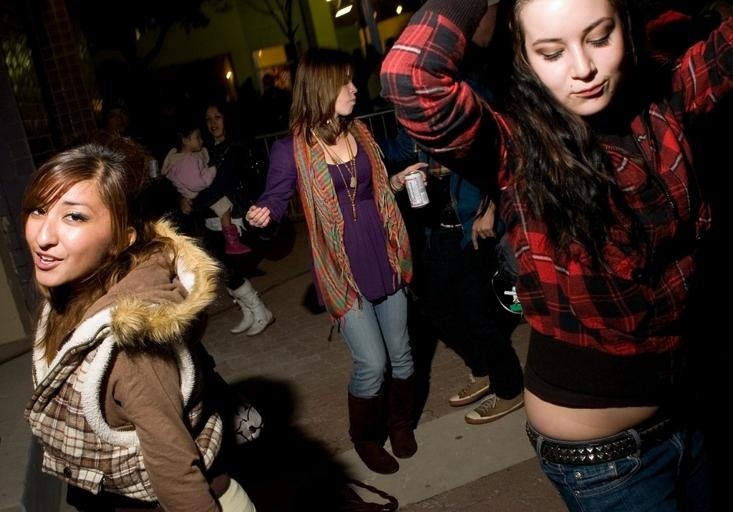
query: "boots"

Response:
[221,224,251,255]
[230,278,275,337]
[225,286,254,334]
[386,370,417,458]
[346,384,399,474]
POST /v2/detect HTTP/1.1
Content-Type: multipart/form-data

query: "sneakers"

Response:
[464,387,524,424]
[447,374,490,407]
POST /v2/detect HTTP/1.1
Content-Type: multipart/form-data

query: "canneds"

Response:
[404,170,429,208]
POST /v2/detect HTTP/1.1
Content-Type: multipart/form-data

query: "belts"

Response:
[526,417,674,466]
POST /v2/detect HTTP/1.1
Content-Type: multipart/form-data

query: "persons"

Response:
[100,24,545,425]
[17,134,259,512]
[375,0,727,509]
[243,44,436,474]
